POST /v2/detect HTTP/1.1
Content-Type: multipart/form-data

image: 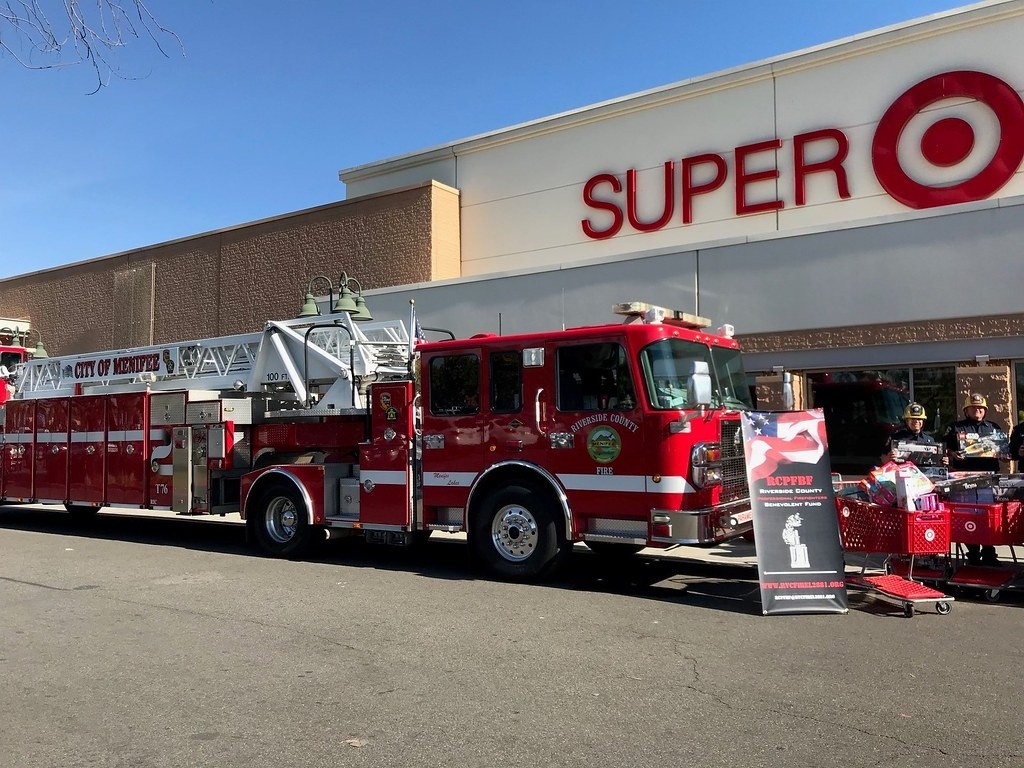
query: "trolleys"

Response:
[832,472,955,617]
[884,496,1024,601]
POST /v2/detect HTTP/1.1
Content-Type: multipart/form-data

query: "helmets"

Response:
[962,393,988,416]
[901,402,928,421]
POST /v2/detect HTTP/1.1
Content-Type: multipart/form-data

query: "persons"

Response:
[1008,423,1024,473]
[881,402,949,467]
[942,393,1012,564]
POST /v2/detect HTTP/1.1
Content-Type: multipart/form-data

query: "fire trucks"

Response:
[0,302,761,581]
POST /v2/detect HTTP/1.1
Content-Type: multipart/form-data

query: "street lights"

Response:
[296,272,374,323]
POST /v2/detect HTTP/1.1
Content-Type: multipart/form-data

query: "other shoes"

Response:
[966,551,981,565]
[981,548,1009,567]
